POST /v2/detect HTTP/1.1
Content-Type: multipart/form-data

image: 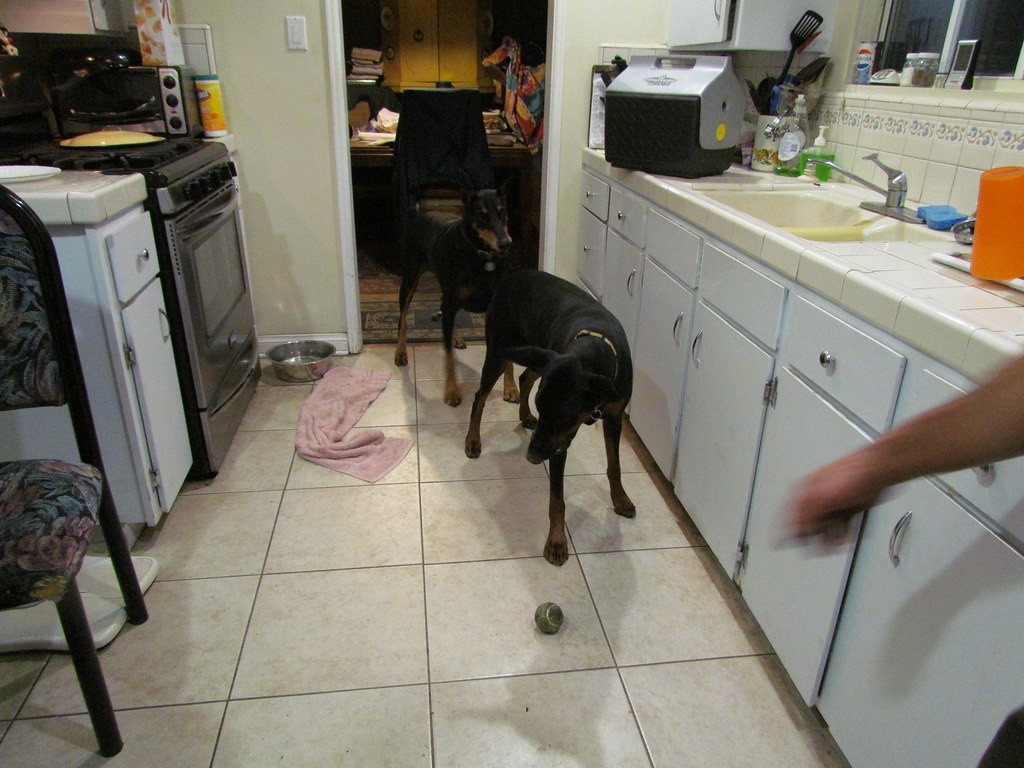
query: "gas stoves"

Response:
[0,52,229,186]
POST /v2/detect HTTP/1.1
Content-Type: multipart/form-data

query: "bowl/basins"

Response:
[265,340,336,383]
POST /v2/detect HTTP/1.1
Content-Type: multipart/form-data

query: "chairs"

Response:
[0,182,149,757]
[391,87,503,270]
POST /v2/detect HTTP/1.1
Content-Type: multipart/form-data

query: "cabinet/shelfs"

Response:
[627,200,793,583]
[667,0,837,52]
[601,181,651,418]
[736,282,1024,768]
[0,202,194,528]
[575,161,610,302]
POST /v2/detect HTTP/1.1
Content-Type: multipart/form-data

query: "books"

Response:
[348,46,384,80]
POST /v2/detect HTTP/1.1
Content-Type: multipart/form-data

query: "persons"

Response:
[786,354,1024,768]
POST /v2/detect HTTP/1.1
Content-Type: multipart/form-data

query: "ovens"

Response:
[138,140,262,482]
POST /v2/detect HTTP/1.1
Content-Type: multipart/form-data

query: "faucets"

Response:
[806,152,927,224]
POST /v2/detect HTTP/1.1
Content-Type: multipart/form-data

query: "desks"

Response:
[350,108,533,267]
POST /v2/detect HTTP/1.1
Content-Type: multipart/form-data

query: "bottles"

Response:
[852,41,877,84]
[900,52,919,85]
[195,75,227,136]
[911,53,940,88]
[774,92,811,179]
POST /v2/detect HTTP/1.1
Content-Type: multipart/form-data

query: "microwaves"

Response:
[53,48,198,140]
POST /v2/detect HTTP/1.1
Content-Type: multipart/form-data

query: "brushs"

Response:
[805,69,826,114]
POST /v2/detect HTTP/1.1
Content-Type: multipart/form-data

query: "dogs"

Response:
[465,267,636,565]
[394,172,518,408]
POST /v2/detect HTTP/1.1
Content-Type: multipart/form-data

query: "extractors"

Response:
[1,0,129,41]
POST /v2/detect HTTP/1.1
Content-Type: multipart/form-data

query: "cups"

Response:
[751,114,793,172]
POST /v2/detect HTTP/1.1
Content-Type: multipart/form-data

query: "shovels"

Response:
[776,10,823,88]
[786,30,823,75]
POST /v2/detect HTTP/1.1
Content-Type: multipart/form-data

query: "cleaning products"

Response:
[773,94,836,183]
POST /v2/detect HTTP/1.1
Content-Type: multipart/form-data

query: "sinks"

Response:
[693,188,959,232]
[777,223,954,243]
